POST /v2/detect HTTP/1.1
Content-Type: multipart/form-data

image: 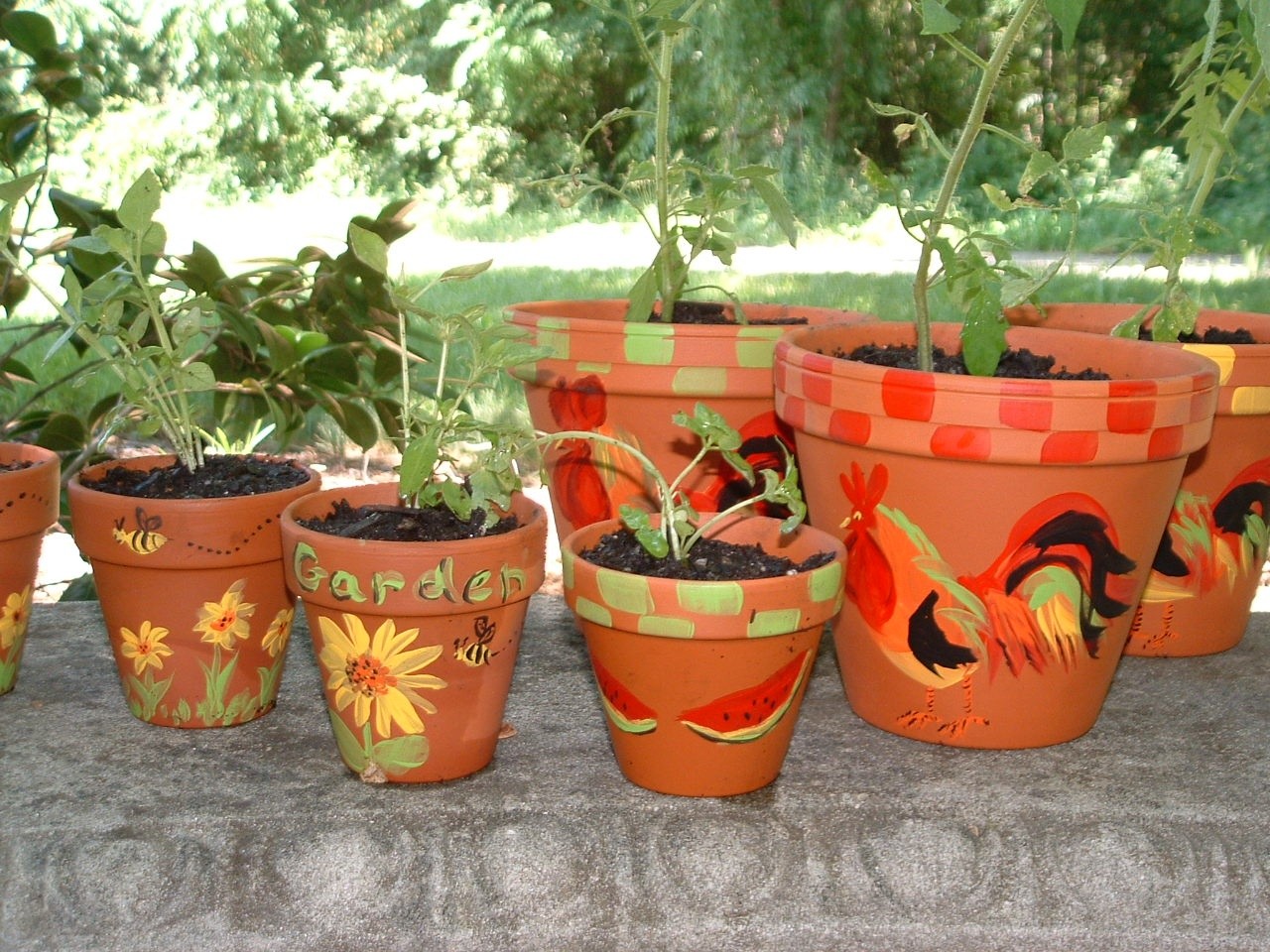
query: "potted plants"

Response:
[1,0,1270,799]
